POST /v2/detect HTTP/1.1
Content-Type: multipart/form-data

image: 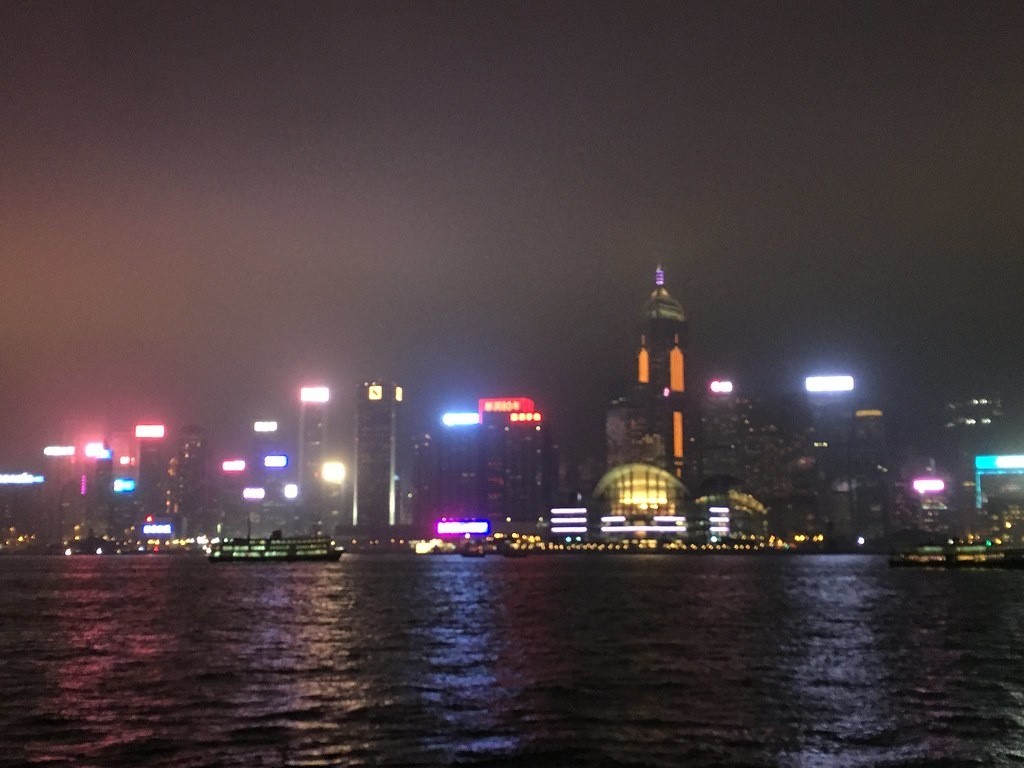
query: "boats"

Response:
[207,516,346,562]
[500,547,527,558]
[888,538,1024,569]
[458,542,486,558]
